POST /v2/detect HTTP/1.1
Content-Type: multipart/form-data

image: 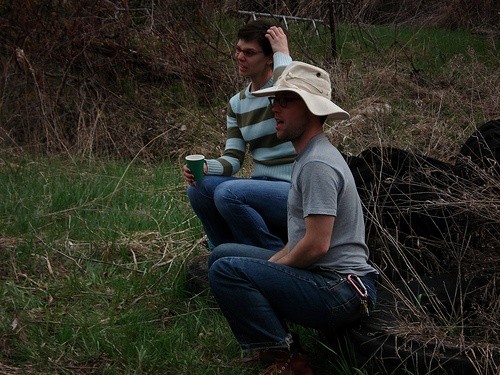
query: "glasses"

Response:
[267,97,303,109]
[232,44,263,57]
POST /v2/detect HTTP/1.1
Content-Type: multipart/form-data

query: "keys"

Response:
[359,293,376,316]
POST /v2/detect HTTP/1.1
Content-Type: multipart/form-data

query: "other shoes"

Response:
[232,332,312,375]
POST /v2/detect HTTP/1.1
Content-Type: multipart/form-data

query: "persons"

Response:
[183,18,305,253]
[206,59,375,375]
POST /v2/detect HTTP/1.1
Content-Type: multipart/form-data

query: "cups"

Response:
[184,154,205,182]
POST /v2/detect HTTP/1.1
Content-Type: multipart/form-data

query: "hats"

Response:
[252,61,349,120]
